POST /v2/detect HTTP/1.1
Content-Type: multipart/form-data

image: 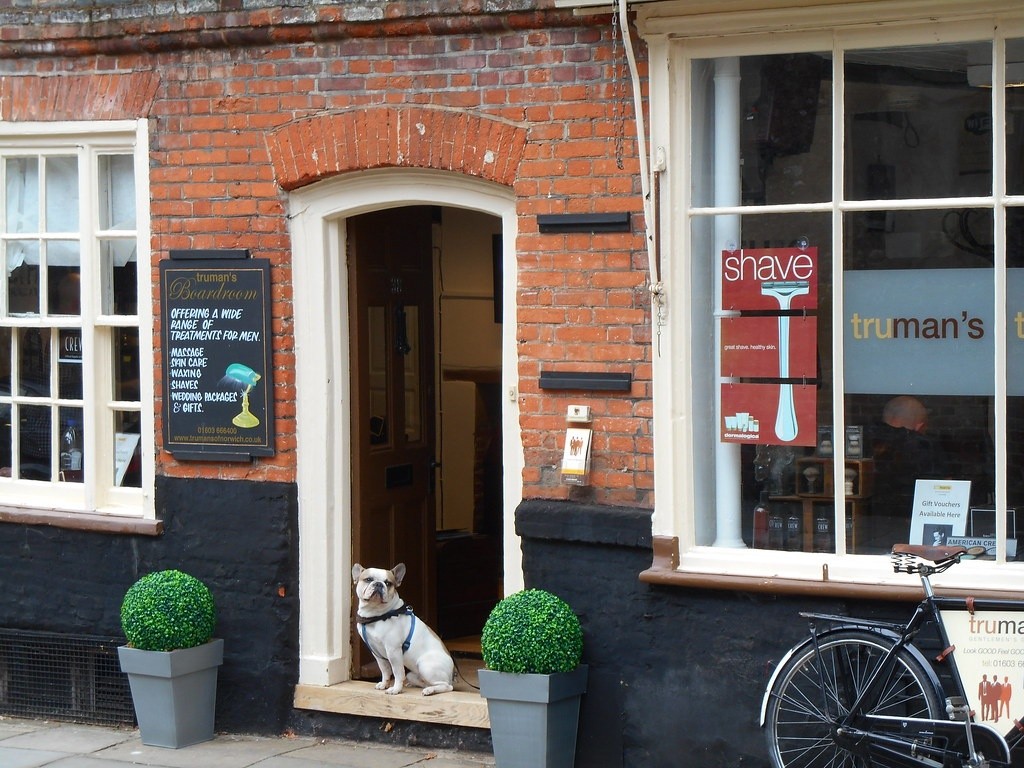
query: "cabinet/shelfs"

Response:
[769,454,876,555]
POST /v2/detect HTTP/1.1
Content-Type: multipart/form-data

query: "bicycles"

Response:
[758,542,1024,768]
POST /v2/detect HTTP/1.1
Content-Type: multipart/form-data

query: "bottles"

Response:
[767,508,853,555]
[60,420,82,482]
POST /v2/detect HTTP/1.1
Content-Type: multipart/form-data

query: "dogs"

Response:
[351,563,455,696]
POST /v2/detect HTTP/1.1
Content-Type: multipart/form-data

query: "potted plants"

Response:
[475,588,588,768]
[117,569,224,750]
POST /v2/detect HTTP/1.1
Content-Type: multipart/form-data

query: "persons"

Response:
[19,273,83,460]
[933,527,946,546]
[864,395,988,517]
